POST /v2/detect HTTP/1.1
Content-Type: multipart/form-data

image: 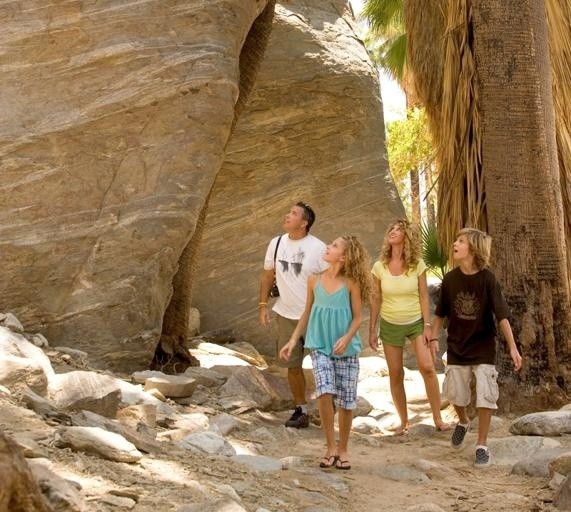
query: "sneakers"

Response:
[285,406,309,429]
[452,420,471,448]
[474,448,490,468]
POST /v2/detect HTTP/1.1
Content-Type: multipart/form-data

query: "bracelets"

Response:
[258,302,268,307]
[424,321,432,326]
[431,337,440,342]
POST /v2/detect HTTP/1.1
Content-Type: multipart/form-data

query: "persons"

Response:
[277,232,374,468]
[368,215,458,433]
[430,227,526,470]
[258,200,328,428]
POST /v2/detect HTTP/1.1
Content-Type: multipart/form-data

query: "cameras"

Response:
[269,286,279,298]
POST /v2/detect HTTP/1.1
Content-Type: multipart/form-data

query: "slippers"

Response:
[336,457,351,470]
[319,456,336,469]
[393,424,451,437]
